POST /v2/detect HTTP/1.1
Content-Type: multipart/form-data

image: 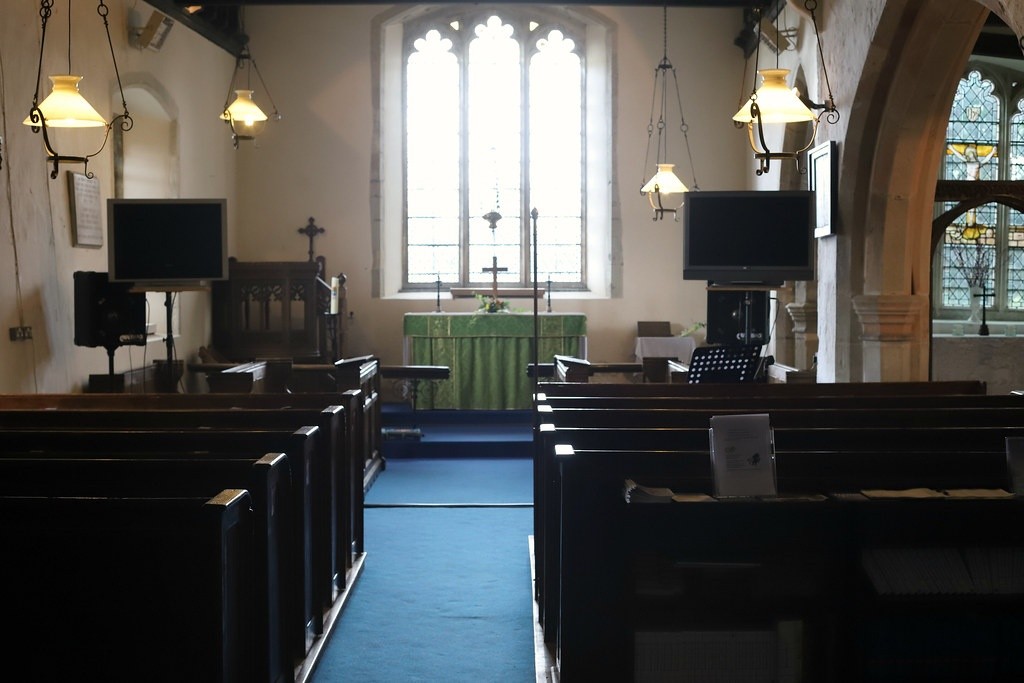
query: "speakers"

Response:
[73,271,147,348]
[706,288,770,345]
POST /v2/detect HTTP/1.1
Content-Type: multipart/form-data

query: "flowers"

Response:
[475,293,508,313]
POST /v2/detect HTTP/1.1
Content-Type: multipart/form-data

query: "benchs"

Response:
[533,382,1024,683]
[188,364,452,428]
[0,355,386,683]
[526,361,643,382]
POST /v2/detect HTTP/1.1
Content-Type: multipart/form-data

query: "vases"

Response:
[967,287,983,323]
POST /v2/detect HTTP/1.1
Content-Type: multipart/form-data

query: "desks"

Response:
[403,311,587,411]
[932,333,1024,393]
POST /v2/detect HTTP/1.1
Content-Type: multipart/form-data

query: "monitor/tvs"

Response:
[683,190,816,279]
[107,198,228,283]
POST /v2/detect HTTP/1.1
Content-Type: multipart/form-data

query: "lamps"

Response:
[733,0,840,175]
[638,1,699,221]
[23,0,133,179]
[220,1,282,151]
